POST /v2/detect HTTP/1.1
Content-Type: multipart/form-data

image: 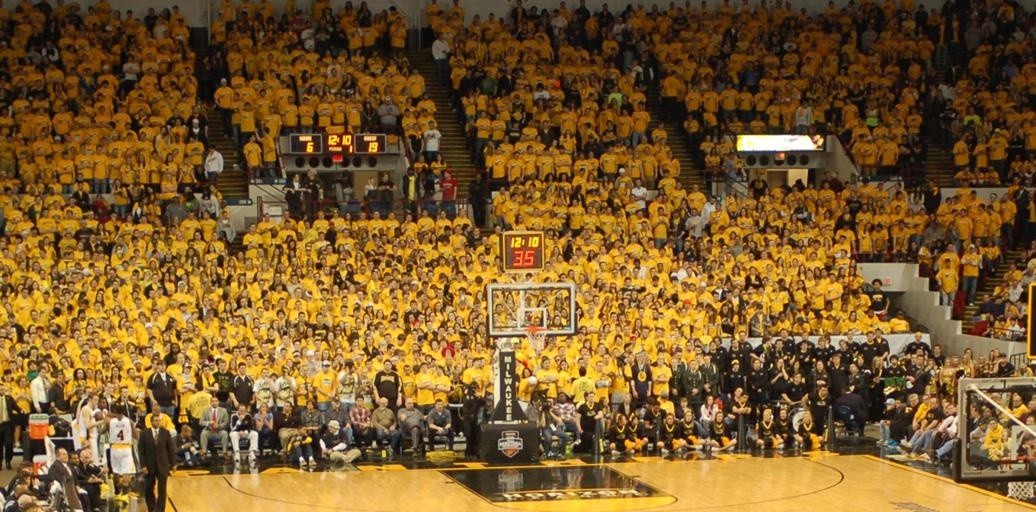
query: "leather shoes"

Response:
[223,456,231,461]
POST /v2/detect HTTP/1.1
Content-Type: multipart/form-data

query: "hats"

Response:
[327,420,339,430]
[883,399,895,405]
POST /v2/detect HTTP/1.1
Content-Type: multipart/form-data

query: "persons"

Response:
[80,393,176,511]
[1,449,102,512]
[0,1,1035,469]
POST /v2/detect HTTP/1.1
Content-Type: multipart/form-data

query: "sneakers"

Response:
[233,454,239,462]
[876,438,936,463]
[308,461,316,466]
[249,454,256,461]
[300,461,306,466]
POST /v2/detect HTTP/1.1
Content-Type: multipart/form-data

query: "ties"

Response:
[211,412,216,433]
[154,431,157,447]
[42,380,47,394]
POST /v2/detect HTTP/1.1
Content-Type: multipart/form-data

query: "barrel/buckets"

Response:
[421,200,437,216]
[347,199,362,220]
[27,414,52,440]
[440,200,458,218]
